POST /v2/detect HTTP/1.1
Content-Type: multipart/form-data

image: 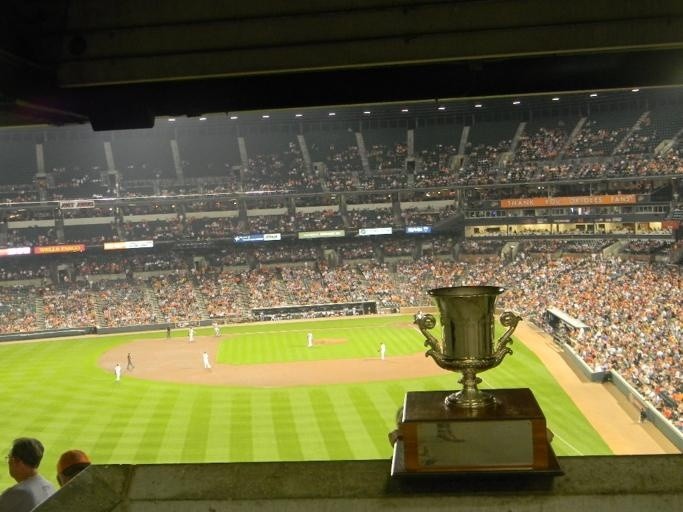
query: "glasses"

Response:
[4,455,18,458]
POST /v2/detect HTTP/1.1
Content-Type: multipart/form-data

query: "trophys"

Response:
[390,285,563,490]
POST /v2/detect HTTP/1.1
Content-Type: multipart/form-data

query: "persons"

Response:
[0,437,57,512]
[56,449,91,487]
[1,119,682,436]
